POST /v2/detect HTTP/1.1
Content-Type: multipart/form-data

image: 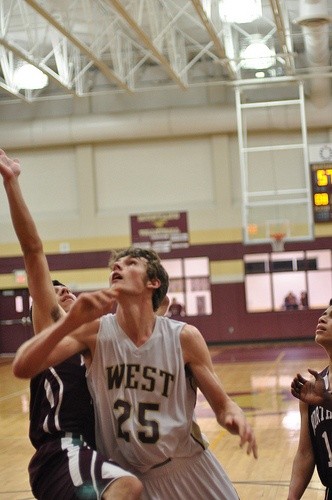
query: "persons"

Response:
[158,293,332,500]
[0,148,147,500]
[13,247,260,500]
